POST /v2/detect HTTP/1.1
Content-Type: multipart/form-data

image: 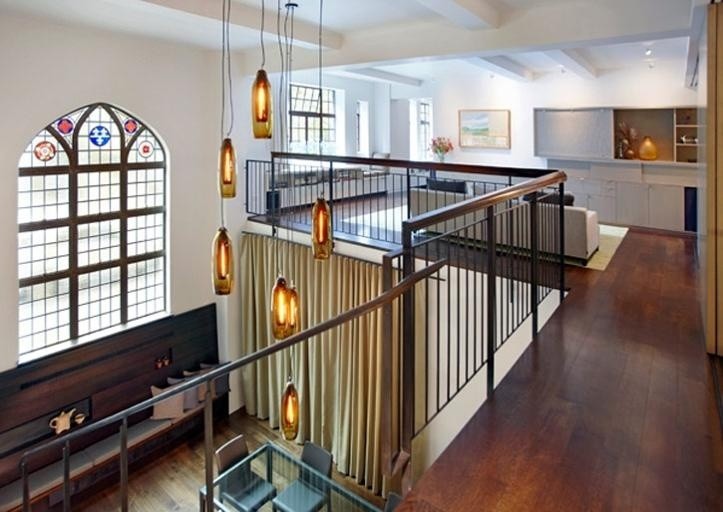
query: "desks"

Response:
[199,442,383,511]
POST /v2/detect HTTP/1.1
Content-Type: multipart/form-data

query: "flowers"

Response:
[430,137,453,156]
[616,118,637,147]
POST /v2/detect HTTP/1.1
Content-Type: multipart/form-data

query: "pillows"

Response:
[522,188,574,206]
[425,177,467,194]
[369,152,390,172]
[149,362,230,420]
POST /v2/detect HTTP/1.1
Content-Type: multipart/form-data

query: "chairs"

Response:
[382,491,402,511]
[214,434,276,511]
[271,439,332,511]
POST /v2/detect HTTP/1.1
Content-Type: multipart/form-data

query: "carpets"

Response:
[340,204,630,273]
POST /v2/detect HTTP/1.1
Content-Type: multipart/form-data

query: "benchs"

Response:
[0,401,208,510]
[261,161,388,209]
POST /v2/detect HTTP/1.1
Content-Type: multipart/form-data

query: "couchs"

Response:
[407,178,599,266]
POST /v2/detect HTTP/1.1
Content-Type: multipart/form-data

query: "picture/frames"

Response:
[458,109,511,150]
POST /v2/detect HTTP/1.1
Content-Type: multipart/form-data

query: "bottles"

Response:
[617,138,623,158]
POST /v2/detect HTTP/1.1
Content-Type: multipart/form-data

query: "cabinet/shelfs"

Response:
[546,158,700,235]
[673,105,698,162]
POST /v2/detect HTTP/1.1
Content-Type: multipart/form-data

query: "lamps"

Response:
[212,3,331,441]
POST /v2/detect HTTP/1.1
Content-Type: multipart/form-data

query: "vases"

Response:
[437,156,444,163]
[637,135,659,162]
[623,145,636,159]
